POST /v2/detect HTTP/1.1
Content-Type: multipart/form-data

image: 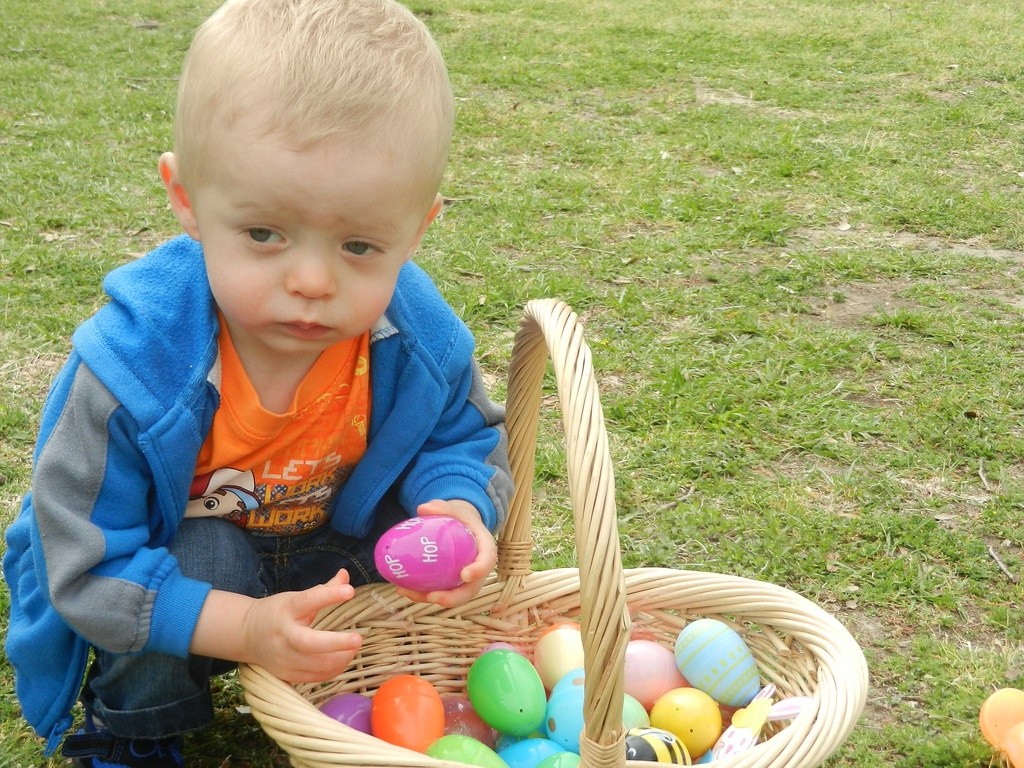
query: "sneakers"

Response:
[63,711,184,768]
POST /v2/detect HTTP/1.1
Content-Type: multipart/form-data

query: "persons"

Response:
[2,0,516,768]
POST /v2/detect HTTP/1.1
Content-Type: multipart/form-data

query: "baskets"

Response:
[237,299,868,768]
